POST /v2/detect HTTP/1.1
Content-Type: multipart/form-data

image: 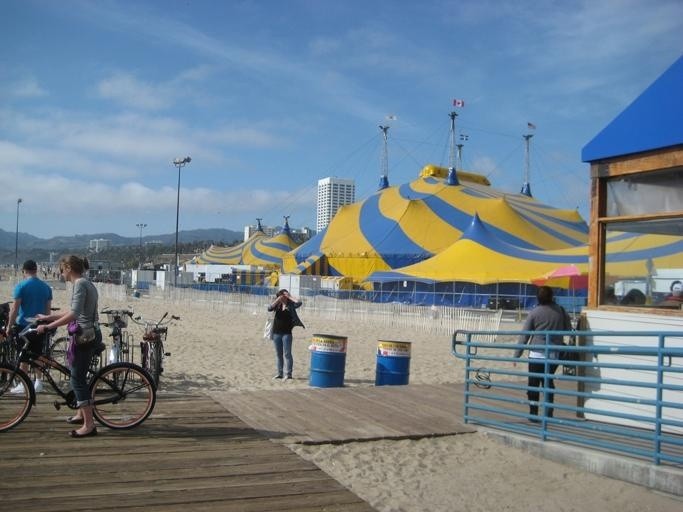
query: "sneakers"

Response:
[272,375,292,381]
[10,382,26,394]
[33,381,43,392]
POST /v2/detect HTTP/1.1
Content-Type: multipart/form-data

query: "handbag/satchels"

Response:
[559,342,579,361]
[264,318,274,340]
[75,327,95,345]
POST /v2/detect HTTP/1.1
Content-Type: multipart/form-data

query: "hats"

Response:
[276,289,289,298]
[22,259,37,270]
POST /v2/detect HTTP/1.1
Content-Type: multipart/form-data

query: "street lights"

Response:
[172,156,191,280]
[135,224,146,248]
[15,199,22,271]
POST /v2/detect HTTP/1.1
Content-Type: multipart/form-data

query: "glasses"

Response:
[60,269,66,274]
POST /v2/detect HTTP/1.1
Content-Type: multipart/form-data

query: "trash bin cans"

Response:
[488,298,517,310]
[133,289,140,298]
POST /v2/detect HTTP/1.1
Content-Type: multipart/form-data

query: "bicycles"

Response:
[0,301,179,431]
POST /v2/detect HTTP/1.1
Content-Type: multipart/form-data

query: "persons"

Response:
[35,255,102,437]
[267,289,302,381]
[514,286,571,422]
[6,260,53,393]
[604,287,646,306]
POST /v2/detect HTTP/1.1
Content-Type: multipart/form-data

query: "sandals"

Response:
[69,426,97,438]
[67,416,83,424]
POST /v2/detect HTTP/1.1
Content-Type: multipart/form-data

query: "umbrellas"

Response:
[531,264,622,319]
